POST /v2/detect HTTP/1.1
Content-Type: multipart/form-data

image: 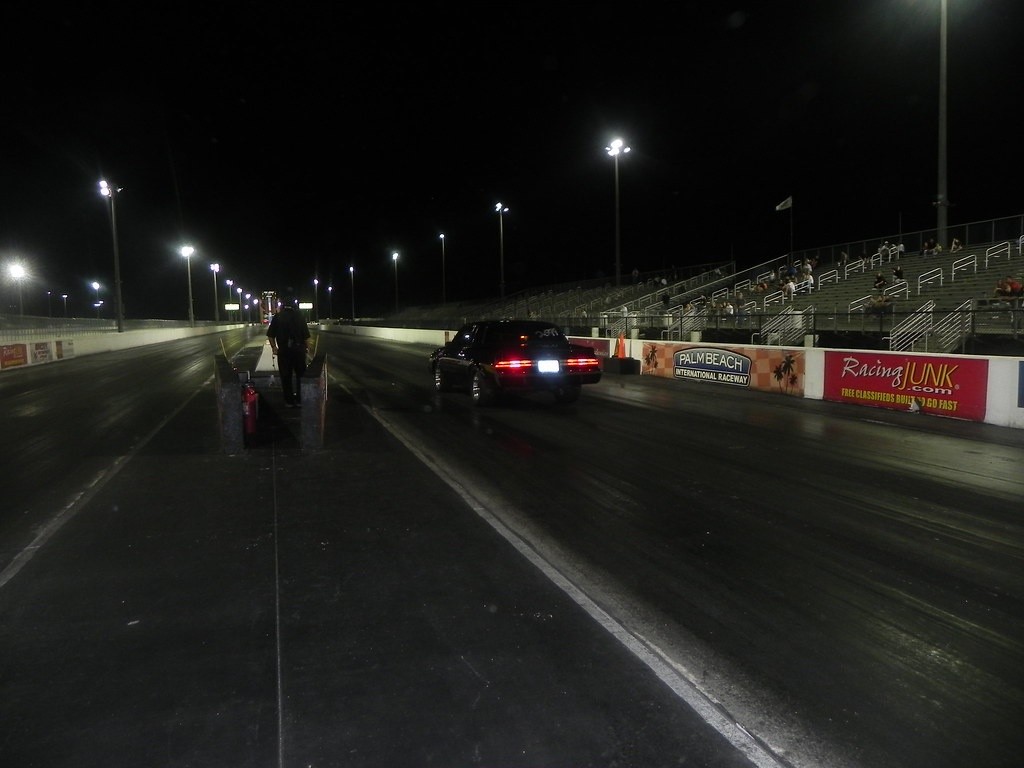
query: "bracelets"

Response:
[305,347,309,351]
[272,345,275,348]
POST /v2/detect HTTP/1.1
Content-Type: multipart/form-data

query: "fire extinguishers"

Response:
[242,381,257,435]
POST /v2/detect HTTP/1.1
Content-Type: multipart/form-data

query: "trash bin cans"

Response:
[792,311,802,329]
[768,333,779,345]
[591,327,599,337]
[663,314,672,327]
[691,330,702,342]
[631,329,639,339]
[805,334,820,347]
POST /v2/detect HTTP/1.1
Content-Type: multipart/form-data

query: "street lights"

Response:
[92,281,99,319]
[210,263,220,326]
[226,280,233,323]
[237,288,243,323]
[10,264,23,317]
[605,138,631,289]
[439,233,446,309]
[62,295,67,318]
[495,202,510,298]
[313,279,319,323]
[47,291,50,317]
[392,252,399,317]
[350,266,355,322]
[182,246,194,329]
[99,180,124,332]
[327,286,333,317]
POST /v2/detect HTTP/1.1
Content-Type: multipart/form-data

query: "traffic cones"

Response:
[617,333,626,359]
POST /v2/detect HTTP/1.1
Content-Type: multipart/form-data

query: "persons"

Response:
[892,265,903,284]
[919,238,942,256]
[952,238,961,249]
[838,251,847,266]
[866,295,894,316]
[751,282,767,294]
[632,268,639,283]
[679,284,686,294]
[663,292,669,306]
[878,241,905,260]
[872,273,885,290]
[267,298,311,407]
[684,292,746,314]
[620,305,627,316]
[769,256,819,296]
[582,309,586,317]
[715,268,722,275]
[990,276,1021,304]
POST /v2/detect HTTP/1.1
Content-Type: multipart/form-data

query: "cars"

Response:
[428,320,602,409]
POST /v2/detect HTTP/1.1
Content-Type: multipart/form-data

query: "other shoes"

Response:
[296,399,303,408]
[287,397,295,408]
